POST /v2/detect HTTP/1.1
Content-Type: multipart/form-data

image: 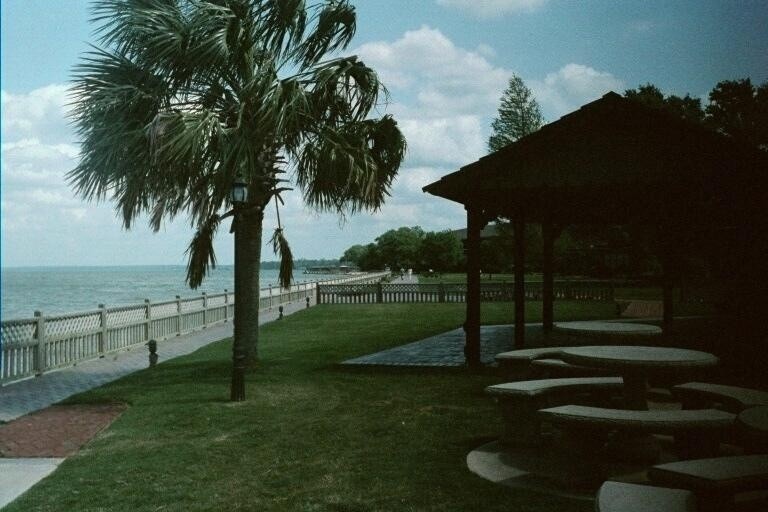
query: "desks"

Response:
[563,346,720,374]
[555,322,662,344]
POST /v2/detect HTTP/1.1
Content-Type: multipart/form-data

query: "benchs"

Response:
[647,452,765,511]
[596,481,694,511]
[673,379,766,436]
[491,345,622,373]
[538,403,736,438]
[488,376,626,416]
